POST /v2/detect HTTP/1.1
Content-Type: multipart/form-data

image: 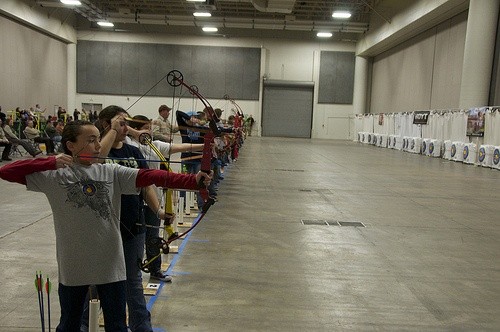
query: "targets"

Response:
[450,144,456,157]
[463,146,468,159]
[493,149,499,164]
[420,141,445,155]
[478,148,485,162]
[358,134,414,149]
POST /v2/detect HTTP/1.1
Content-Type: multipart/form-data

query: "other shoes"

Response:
[151,270,172,281]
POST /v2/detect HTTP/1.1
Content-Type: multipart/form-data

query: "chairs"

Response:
[23,130,43,157]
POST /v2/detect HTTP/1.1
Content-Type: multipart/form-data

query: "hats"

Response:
[158,105,171,111]
[186,112,200,116]
[216,108,224,113]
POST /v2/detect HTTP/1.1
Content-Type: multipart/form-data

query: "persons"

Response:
[0,120,214,332]
[0,103,255,332]
